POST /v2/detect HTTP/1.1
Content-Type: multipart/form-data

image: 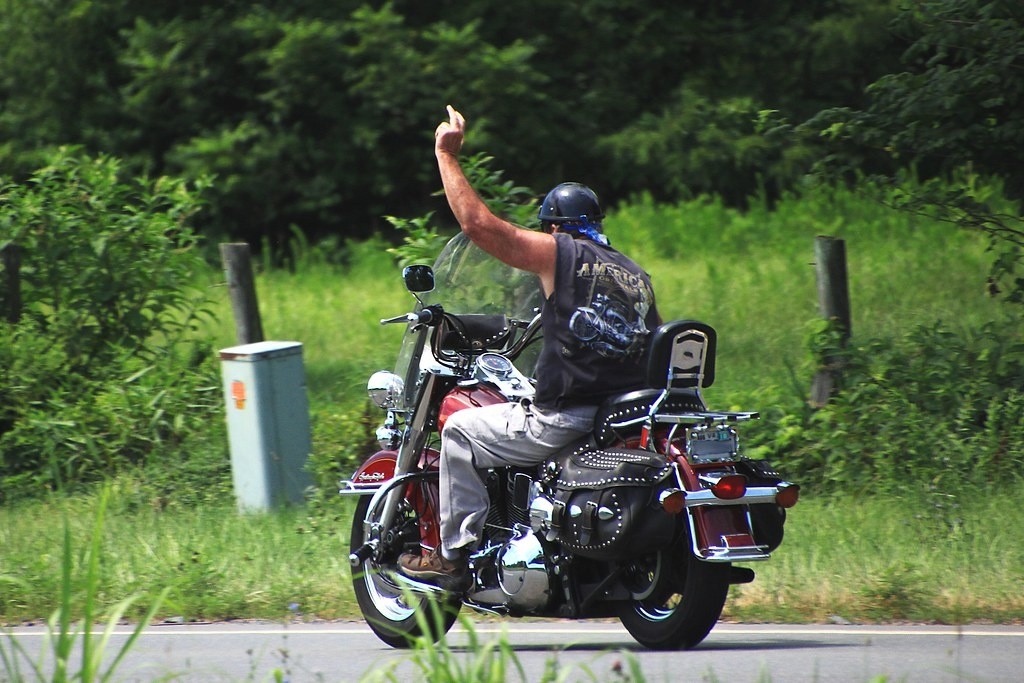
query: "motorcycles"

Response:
[337,221,801,652]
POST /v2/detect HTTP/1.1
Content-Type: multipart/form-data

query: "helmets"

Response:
[537,182,606,222]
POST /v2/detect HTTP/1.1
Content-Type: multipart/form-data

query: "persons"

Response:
[397,104,663,592]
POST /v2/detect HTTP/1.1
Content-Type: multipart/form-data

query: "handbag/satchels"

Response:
[547,444,682,561]
[738,457,786,555]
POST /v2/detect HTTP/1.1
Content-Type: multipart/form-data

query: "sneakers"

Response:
[397,544,473,591]
